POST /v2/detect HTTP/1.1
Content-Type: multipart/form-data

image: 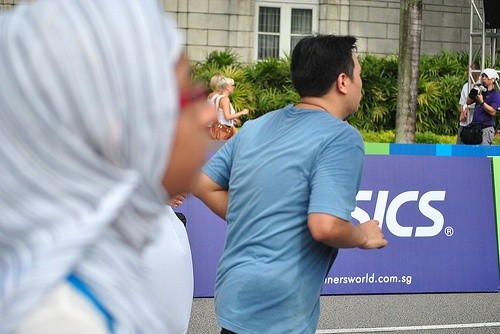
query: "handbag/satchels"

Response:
[209,121,234,141]
[461,124,483,144]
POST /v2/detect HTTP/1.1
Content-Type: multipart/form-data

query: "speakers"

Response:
[483,0,500,29]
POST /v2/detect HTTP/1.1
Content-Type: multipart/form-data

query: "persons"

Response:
[206,74,249,141]
[0,0,218,334]
[455,64,482,144]
[465,68,500,145]
[187,32,388,334]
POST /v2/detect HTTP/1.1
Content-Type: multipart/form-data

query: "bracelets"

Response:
[481,101,484,105]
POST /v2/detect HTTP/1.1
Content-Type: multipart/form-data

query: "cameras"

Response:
[468,83,488,100]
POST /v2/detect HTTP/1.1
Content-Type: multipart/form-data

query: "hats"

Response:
[481,68,499,80]
